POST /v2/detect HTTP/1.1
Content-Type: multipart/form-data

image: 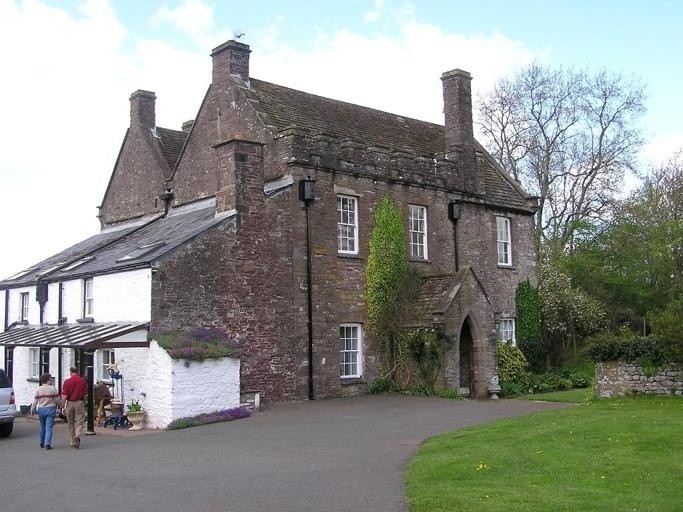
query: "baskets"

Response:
[94,381,113,406]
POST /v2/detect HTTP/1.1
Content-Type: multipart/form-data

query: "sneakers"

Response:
[40,442,45,449]
[46,444,53,450]
[71,437,80,449]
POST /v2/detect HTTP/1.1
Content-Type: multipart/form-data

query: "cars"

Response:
[0,368,15,437]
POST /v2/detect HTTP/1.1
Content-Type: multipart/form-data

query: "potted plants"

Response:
[124,400,147,429]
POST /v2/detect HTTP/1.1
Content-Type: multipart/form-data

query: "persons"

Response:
[61,366,88,449]
[30,373,62,450]
